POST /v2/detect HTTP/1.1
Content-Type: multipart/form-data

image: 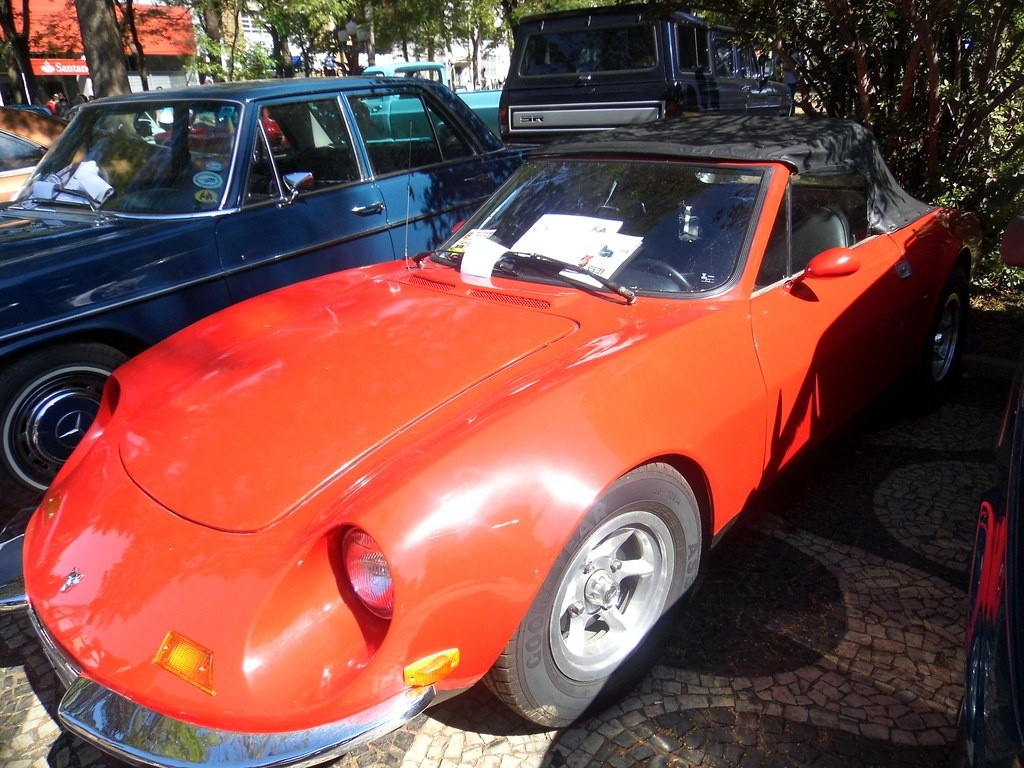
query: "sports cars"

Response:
[20,109,972,768]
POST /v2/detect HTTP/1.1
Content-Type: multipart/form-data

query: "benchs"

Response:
[281,138,456,176]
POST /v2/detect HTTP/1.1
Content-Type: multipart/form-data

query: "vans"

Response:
[498,1,795,150]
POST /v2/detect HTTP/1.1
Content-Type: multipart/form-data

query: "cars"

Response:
[0,73,521,510]
[0,57,505,227]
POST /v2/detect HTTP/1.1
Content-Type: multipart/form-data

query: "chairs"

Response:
[608,181,685,232]
[707,199,849,283]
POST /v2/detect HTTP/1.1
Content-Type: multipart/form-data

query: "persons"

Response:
[30,86,179,136]
